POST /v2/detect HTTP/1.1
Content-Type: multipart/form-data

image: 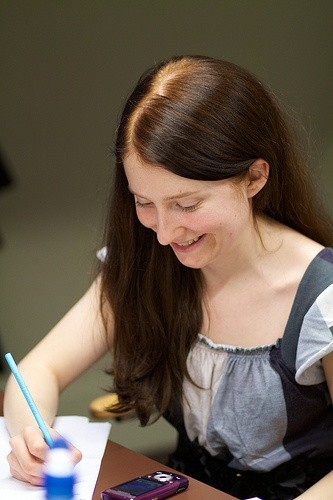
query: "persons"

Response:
[3,56,333,500]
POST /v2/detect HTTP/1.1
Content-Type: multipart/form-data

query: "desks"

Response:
[1,389,244,500]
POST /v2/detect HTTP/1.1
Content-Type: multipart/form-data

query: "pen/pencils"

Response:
[5,352,56,449]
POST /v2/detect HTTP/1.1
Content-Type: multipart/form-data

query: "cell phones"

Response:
[101,470,189,500]
